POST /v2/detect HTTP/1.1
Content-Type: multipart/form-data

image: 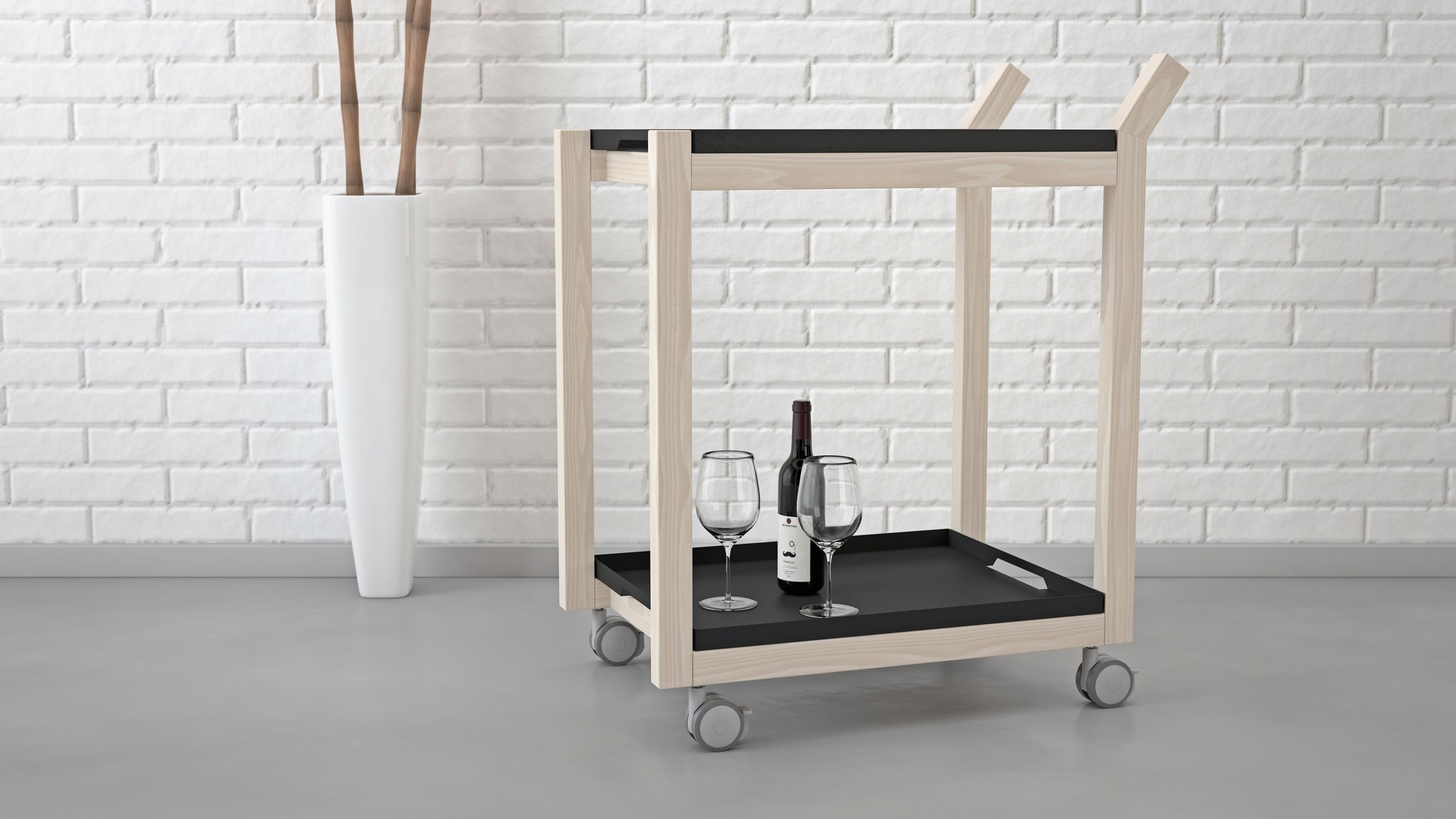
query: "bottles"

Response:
[777,399,822,597]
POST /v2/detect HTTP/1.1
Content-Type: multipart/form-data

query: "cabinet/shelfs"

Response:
[550,46,1189,752]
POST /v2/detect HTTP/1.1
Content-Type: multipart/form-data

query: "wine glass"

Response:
[799,450,860,619]
[696,450,757,613]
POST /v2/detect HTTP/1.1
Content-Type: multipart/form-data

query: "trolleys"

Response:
[554,46,1193,753]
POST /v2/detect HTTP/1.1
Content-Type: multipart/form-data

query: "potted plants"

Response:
[323,0,433,600]
